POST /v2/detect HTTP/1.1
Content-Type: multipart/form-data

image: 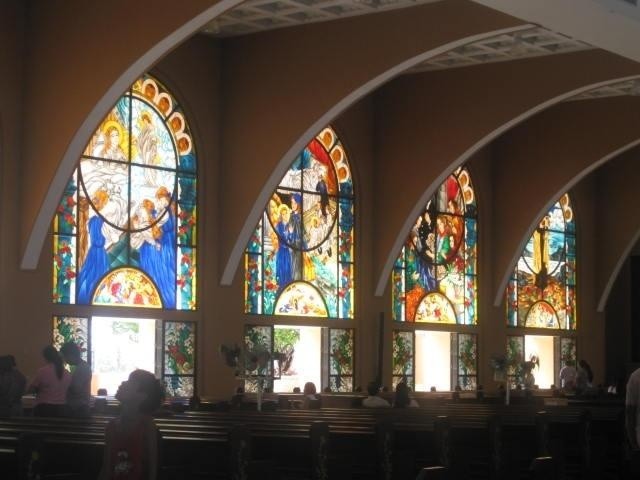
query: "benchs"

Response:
[0,389,624,480]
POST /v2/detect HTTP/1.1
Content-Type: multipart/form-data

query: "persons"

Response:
[98,371,164,479]
[391,382,420,408]
[362,382,392,409]
[26,345,74,419]
[58,340,92,415]
[295,383,319,410]
[0,356,20,418]
[624,367,640,466]
[558,358,577,393]
[577,360,594,388]
[7,353,28,399]
